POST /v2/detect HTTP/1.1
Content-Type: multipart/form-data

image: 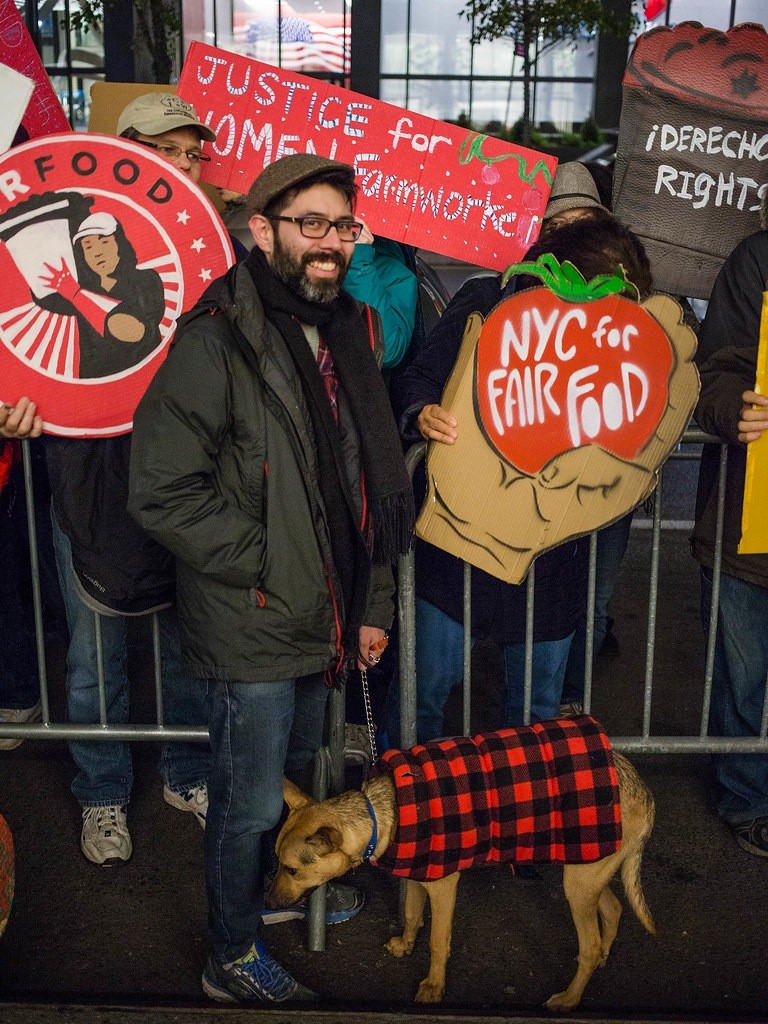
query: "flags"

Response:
[246,13,351,73]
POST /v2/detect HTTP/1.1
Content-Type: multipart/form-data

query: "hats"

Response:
[541,160,617,220]
[246,152,357,215]
[116,92,217,145]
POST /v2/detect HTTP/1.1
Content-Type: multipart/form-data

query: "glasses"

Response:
[128,138,212,168]
[263,215,364,246]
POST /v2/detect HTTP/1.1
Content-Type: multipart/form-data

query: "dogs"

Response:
[264,713,663,1012]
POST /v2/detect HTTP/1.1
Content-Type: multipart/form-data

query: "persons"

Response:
[0,94,232,869]
[374,217,655,755]
[691,180,768,859]
[339,212,418,385]
[0,434,49,754]
[124,154,416,1006]
[536,159,636,721]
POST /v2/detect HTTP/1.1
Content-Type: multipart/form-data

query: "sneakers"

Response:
[163,781,209,833]
[196,937,324,1007]
[76,803,137,870]
[258,877,368,927]
[732,814,768,858]
